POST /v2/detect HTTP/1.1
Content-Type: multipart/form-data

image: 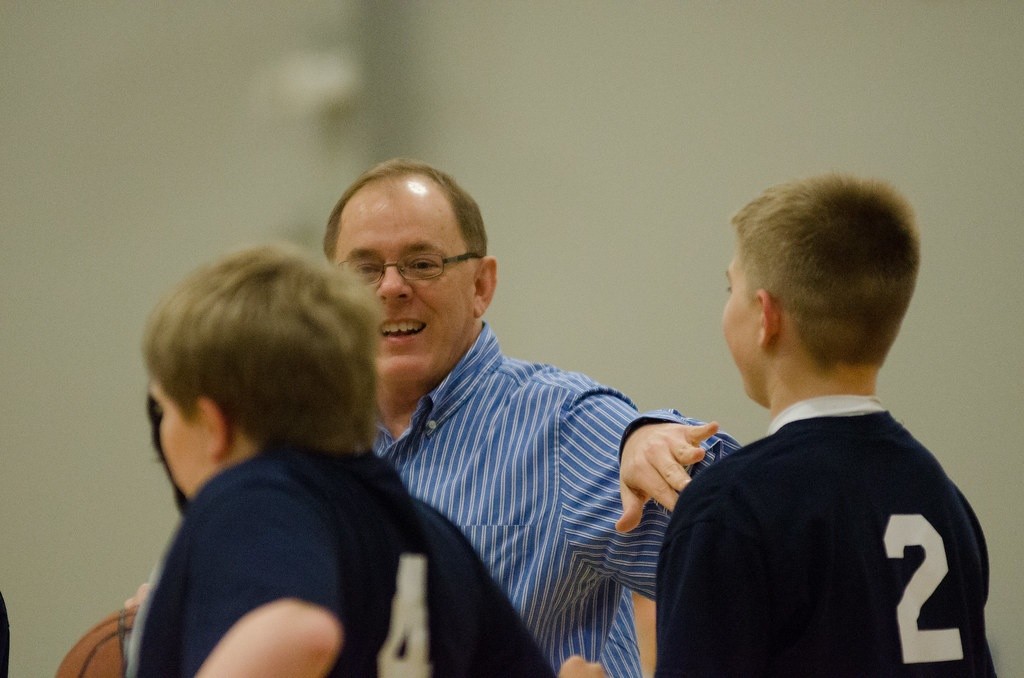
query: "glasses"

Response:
[339,251,483,285]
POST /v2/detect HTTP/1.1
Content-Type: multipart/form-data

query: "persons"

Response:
[325,162,744,678]
[652,171,1001,677]
[124,247,610,678]
